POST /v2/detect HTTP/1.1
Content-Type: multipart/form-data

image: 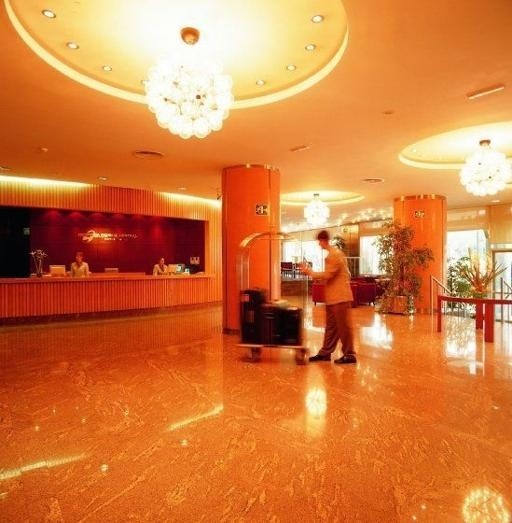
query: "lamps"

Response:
[142,26,235,141]
[458,136,511,196]
[303,192,331,227]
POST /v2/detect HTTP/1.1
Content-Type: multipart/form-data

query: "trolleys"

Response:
[237,230,309,365]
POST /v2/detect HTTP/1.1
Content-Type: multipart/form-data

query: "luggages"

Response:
[241,287,302,345]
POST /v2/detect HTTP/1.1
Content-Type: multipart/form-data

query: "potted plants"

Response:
[30,246,48,277]
[371,216,437,316]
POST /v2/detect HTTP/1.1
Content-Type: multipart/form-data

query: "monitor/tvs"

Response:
[185,267,190,275]
[177,265,182,273]
[49,264,65,276]
[165,264,177,273]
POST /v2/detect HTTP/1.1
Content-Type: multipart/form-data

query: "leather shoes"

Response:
[309,354,331,361]
[334,356,356,363]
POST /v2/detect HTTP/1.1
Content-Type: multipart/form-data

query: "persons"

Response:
[71,252,89,274]
[300,231,360,365]
[153,257,169,276]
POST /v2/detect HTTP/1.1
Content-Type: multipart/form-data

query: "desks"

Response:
[436,290,512,342]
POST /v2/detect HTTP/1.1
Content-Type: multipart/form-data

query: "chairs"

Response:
[281,257,313,280]
[311,275,390,308]
